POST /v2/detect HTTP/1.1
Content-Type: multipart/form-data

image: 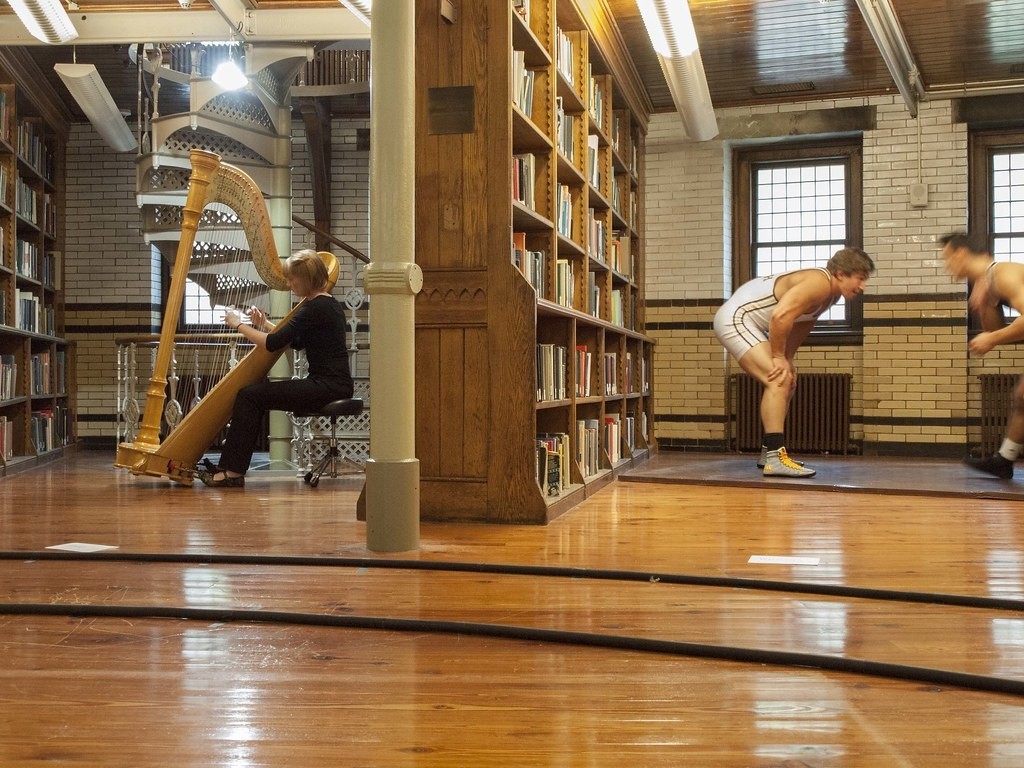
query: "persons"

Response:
[942,231,1024,478]
[713,248,875,478]
[199,248,354,488]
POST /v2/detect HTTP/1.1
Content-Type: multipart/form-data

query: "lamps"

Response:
[211,28,248,90]
[854,0,927,120]
[7,0,79,45]
[53,44,138,152]
[339,0,372,29]
[637,1,719,141]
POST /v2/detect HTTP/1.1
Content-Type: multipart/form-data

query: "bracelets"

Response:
[236,323,244,332]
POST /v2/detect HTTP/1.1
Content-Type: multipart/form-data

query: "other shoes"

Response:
[964,452,1015,480]
[198,469,246,487]
[204,458,221,471]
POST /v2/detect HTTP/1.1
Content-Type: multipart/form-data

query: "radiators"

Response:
[977,373,1021,457]
[726,373,853,457]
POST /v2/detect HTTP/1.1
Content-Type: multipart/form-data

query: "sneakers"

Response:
[757,445,804,468]
[763,446,817,478]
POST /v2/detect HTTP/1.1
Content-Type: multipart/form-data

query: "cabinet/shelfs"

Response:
[0,46,83,477]
[356,0,658,526]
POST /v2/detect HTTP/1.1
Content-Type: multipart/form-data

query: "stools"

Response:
[294,399,367,487]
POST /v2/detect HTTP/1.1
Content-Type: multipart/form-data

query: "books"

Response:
[512,0,649,498]
[0,91,73,463]
